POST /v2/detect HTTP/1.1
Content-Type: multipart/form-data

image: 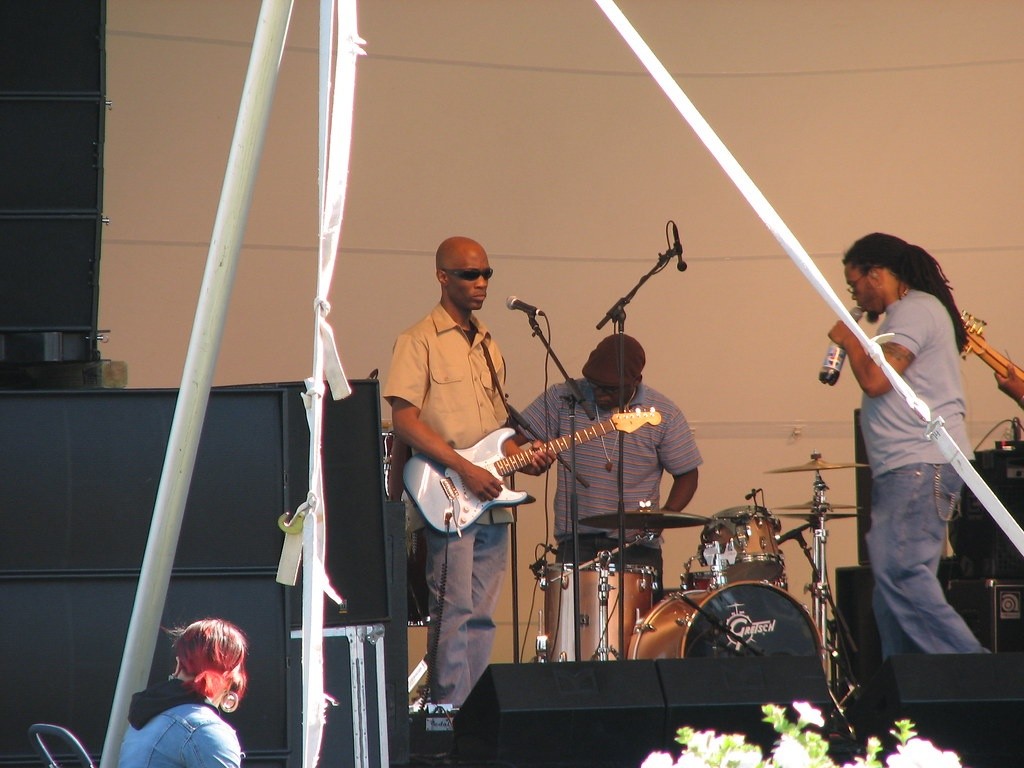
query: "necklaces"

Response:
[898,286,909,300]
[594,404,620,473]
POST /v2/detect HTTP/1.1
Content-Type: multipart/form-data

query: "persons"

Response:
[994,364,1024,410]
[383,236,556,712]
[513,333,704,604]
[118,613,250,768]
[828,233,993,662]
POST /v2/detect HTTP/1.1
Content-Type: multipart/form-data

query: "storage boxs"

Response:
[948,578,1024,654]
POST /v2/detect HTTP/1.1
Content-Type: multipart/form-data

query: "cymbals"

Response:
[769,501,864,522]
[578,509,713,529]
[762,460,872,475]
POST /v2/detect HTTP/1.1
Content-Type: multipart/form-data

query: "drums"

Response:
[699,504,785,584]
[535,563,658,663]
[680,571,788,590]
[627,580,821,660]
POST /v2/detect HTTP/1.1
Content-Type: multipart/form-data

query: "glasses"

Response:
[588,381,631,395]
[847,266,873,298]
[440,267,494,281]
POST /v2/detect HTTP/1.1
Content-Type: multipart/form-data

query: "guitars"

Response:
[402,406,662,535]
[961,309,1024,387]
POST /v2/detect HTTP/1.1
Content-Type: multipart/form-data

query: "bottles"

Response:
[818,339,845,386]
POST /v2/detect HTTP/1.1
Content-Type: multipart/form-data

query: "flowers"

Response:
[638,701,961,768]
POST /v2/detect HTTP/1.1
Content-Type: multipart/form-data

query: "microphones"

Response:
[745,489,760,500]
[506,296,545,316]
[850,307,863,321]
[541,544,558,555]
[673,223,687,271]
[775,523,812,545]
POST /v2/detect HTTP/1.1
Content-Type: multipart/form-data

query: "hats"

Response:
[582,333,645,387]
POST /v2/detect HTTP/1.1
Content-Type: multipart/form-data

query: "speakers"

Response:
[834,561,965,702]
[949,578,1024,653]
[954,484,1024,580]
[839,651,1024,768]
[853,409,879,566]
[452,659,667,768]
[656,652,834,768]
[0,0,107,331]
